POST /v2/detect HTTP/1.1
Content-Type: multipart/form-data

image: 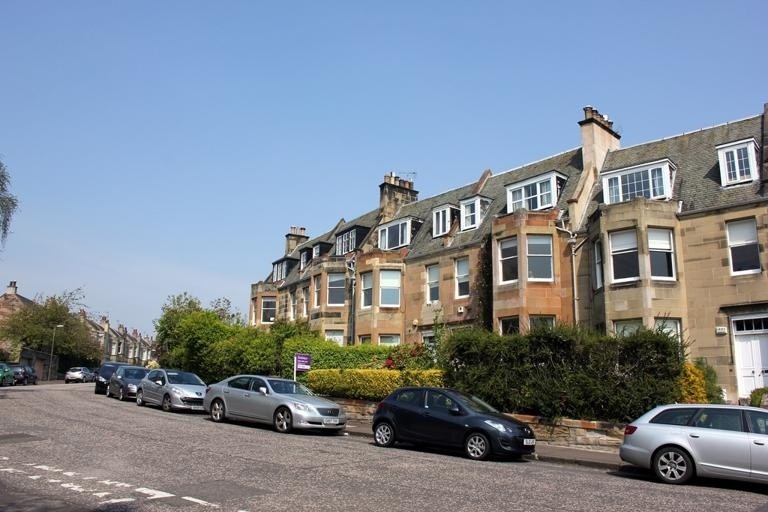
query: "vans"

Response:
[614,399,767,489]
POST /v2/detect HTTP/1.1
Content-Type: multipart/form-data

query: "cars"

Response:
[202,375,349,436]
[1,362,17,386]
[11,365,39,387]
[64,359,151,403]
[370,383,537,463]
[133,364,211,416]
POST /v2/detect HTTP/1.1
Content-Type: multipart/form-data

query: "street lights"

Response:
[45,323,64,382]
